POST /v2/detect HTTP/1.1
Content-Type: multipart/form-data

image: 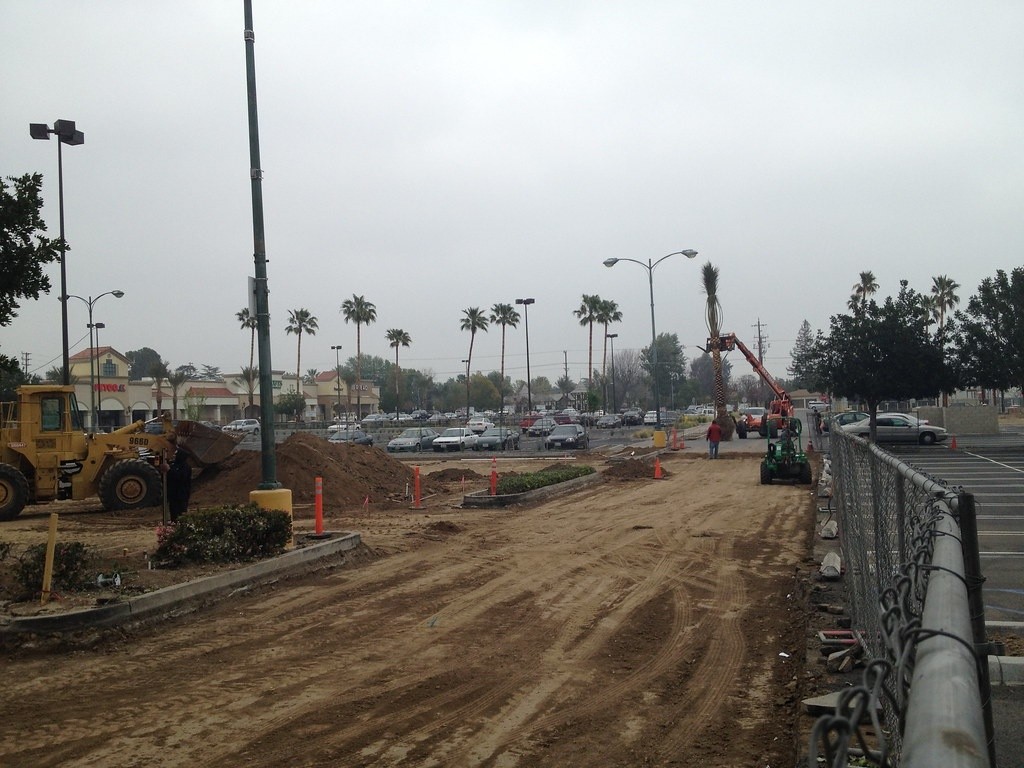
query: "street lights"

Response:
[86,323,105,429]
[29,120,86,384]
[607,334,618,414]
[604,248,698,430]
[462,360,469,376]
[331,345,342,419]
[515,298,535,415]
[57,290,124,427]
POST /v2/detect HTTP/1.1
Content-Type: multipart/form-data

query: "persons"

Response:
[706,421,722,458]
[810,410,823,435]
[154,431,192,526]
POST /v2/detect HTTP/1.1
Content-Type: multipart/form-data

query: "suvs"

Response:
[948,402,975,407]
[144,419,261,435]
[808,401,830,410]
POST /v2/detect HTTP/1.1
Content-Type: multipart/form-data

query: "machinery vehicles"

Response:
[0,384,249,520]
[697,333,803,439]
[760,418,812,485]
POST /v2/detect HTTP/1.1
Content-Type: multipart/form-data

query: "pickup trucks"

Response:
[683,409,718,418]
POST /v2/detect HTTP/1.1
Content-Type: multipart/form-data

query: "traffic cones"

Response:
[950,436,957,448]
[653,458,666,478]
[678,437,687,449]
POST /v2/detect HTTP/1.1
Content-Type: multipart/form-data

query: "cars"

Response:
[545,422,590,449]
[475,426,520,450]
[821,411,949,446]
[527,408,681,430]
[387,427,440,452]
[520,416,558,436]
[465,417,495,432]
[432,427,478,452]
[327,412,373,448]
[362,410,496,420]
